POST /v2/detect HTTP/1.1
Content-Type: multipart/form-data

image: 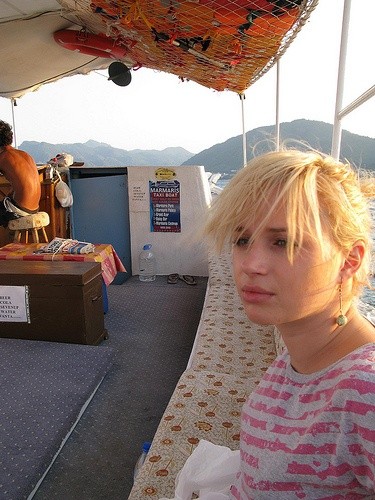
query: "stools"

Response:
[8,211,51,242]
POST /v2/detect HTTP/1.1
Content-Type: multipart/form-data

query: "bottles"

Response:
[134,441,151,484]
[139,244,156,281]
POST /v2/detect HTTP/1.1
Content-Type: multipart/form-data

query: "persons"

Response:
[0,120,41,227]
[198,151,374,500]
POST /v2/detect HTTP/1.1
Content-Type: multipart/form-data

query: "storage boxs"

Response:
[0,260,109,344]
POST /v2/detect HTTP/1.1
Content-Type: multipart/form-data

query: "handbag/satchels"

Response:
[55,168,74,208]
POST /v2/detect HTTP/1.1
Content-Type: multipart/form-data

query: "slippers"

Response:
[167,273,179,284]
[179,274,198,285]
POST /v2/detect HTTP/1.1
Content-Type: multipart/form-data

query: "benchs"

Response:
[128,236,278,500]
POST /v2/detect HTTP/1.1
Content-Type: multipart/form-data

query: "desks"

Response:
[0,239,127,316]
